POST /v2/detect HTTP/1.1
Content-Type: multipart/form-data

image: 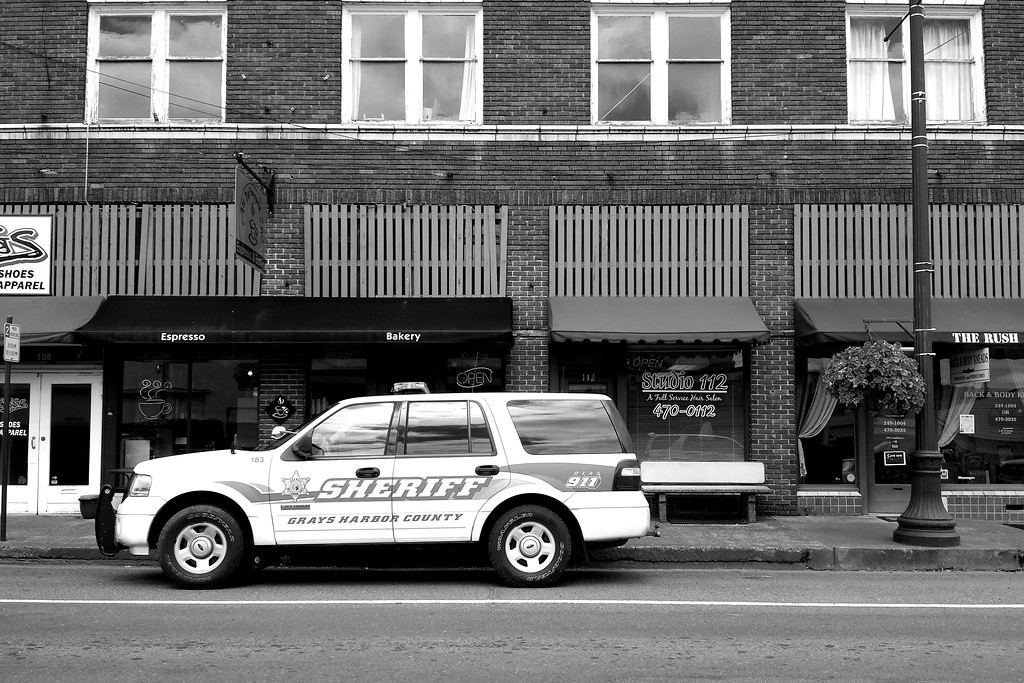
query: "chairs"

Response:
[995,444,1016,483]
[962,451,984,483]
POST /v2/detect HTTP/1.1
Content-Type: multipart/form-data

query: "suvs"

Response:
[92,381,652,589]
[646,431,745,461]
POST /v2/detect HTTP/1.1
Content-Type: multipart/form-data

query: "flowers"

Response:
[822,337,925,420]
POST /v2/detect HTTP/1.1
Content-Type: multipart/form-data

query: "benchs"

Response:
[638,460,771,523]
